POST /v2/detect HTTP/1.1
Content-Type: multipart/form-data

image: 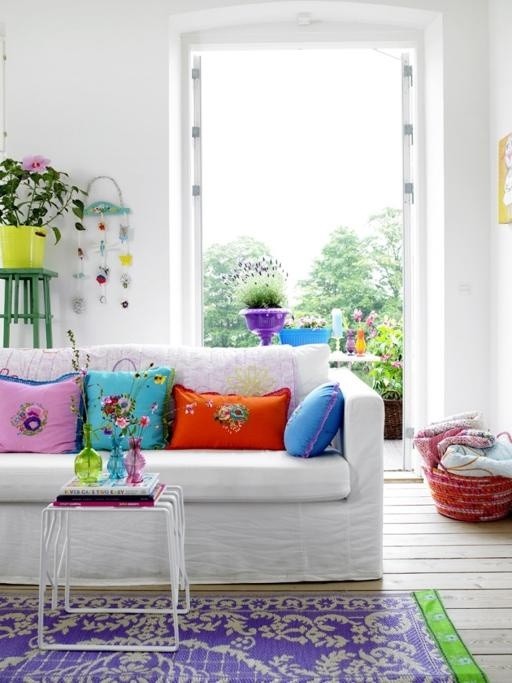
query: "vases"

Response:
[1,225,46,270]
[124,438,145,483]
[74,424,103,485]
[105,434,124,481]
[347,330,355,355]
[356,328,366,355]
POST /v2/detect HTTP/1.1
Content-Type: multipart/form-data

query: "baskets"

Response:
[420,432,512,521]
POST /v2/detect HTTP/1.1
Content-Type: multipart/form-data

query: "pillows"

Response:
[0,370,80,454]
[82,367,171,452]
[170,383,292,453]
[283,381,344,457]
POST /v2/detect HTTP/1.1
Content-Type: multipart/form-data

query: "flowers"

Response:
[111,362,167,476]
[67,328,93,475]
[117,415,149,478]
[0,156,83,226]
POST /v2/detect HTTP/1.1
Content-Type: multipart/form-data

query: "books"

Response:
[53,470,166,507]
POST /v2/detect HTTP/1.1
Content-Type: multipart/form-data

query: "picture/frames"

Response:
[498,135,512,226]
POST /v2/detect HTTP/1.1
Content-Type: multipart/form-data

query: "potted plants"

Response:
[221,255,287,344]
[281,311,331,346]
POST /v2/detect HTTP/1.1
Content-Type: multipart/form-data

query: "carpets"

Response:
[0,592,488,683]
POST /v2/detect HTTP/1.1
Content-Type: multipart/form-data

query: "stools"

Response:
[1,268,59,348]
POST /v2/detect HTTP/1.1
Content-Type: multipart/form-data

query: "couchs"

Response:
[0,344,385,587]
